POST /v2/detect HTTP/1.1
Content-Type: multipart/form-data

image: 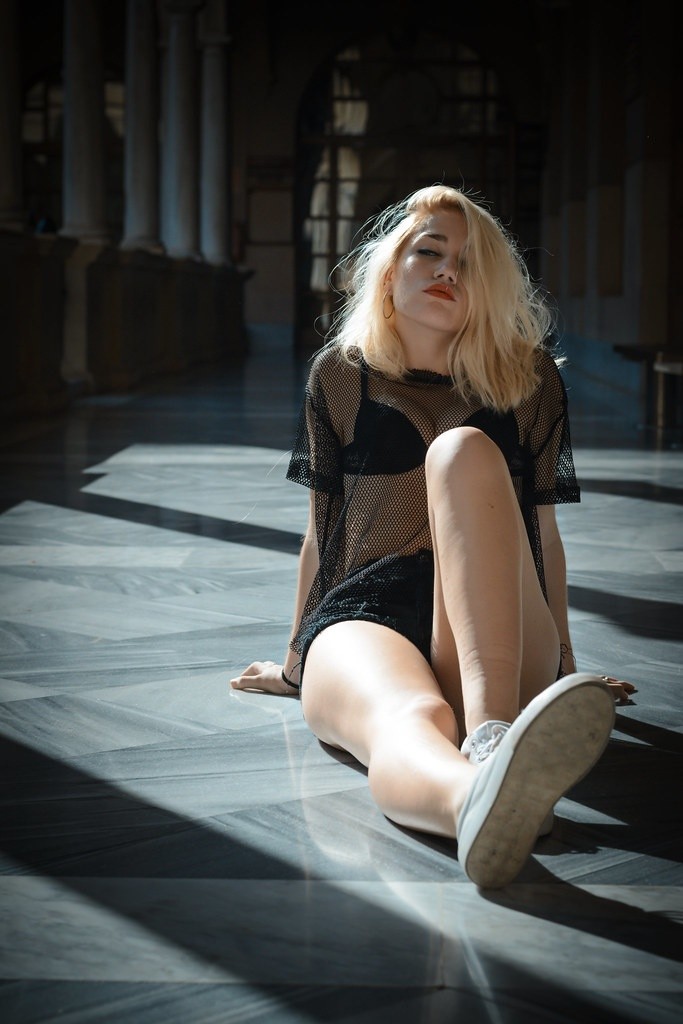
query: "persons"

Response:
[230,185,640,890]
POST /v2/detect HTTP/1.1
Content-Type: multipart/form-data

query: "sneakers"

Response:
[456,672,618,888]
[461,719,554,835]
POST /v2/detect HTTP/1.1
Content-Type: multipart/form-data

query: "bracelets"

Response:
[282,668,301,690]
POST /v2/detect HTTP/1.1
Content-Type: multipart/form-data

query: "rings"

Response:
[601,677,610,683]
